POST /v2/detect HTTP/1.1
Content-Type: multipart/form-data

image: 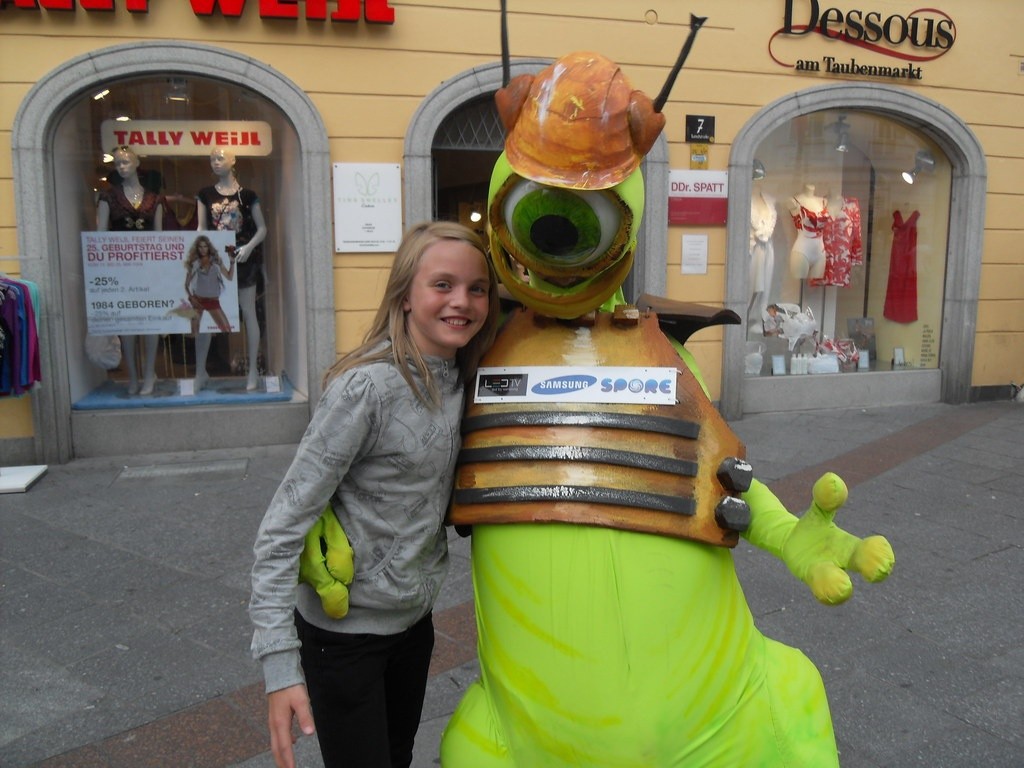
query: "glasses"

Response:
[112,145,128,153]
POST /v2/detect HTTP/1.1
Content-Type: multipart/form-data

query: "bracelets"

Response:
[230,262,235,265]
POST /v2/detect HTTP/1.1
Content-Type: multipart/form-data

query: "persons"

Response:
[96,146,166,394]
[250,220,493,768]
[184,235,235,334]
[762,304,785,338]
[189,146,267,395]
[747,181,863,323]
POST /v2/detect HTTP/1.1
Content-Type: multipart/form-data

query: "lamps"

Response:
[901,152,937,185]
[753,159,766,180]
[824,116,852,153]
[164,80,193,104]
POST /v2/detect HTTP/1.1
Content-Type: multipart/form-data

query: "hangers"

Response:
[0,283,20,295]
[901,202,912,214]
[0,287,16,300]
